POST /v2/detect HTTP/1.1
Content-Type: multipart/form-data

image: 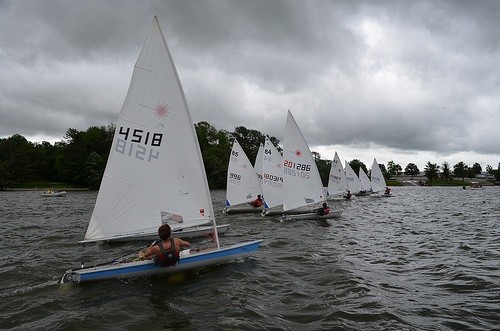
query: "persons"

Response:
[249,194,262,207]
[369,186,372,192]
[384,187,391,194]
[318,203,330,216]
[360,190,366,194]
[463,185,466,189]
[48,187,53,194]
[343,191,351,199]
[419,179,432,185]
[145,224,191,266]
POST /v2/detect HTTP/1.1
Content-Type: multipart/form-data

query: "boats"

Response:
[108,222,231,245]
[463,182,486,191]
[40,190,67,198]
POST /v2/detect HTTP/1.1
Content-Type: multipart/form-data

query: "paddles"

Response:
[112,244,149,263]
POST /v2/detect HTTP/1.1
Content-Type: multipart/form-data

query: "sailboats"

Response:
[221,138,266,216]
[56,14,262,284]
[343,158,394,198]
[259,136,290,217]
[279,110,345,222]
[323,151,356,203]
